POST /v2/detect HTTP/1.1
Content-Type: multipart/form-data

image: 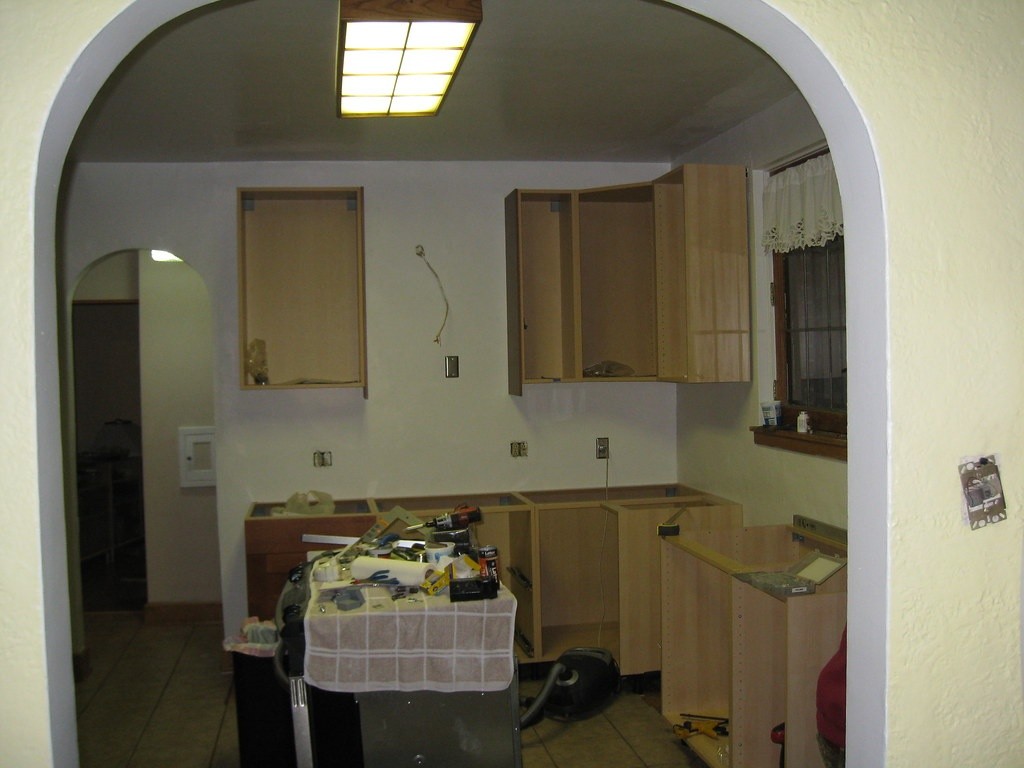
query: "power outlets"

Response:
[313,452,331,466]
[511,442,528,456]
[596,438,609,458]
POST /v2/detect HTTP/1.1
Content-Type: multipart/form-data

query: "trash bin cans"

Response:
[233,650,365,768]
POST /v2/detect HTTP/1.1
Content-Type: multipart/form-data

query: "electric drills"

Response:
[405,504,482,531]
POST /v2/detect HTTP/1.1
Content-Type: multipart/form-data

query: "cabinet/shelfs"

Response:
[505,164,751,396]
[244,484,847,768]
[78,461,146,566]
[303,550,519,768]
[237,186,364,399]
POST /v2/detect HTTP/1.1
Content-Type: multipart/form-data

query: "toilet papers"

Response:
[350,555,437,588]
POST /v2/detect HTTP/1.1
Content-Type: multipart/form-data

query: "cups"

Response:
[761,401,782,426]
[425,542,455,563]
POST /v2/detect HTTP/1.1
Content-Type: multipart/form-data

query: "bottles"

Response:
[425,507,482,530]
[797,410,810,432]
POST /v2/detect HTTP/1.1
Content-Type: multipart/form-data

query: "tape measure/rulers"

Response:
[340,505,441,557]
[656,503,688,536]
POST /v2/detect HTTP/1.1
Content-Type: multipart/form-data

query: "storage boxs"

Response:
[420,555,481,596]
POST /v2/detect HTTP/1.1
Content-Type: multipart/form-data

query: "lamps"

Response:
[336,0,483,119]
